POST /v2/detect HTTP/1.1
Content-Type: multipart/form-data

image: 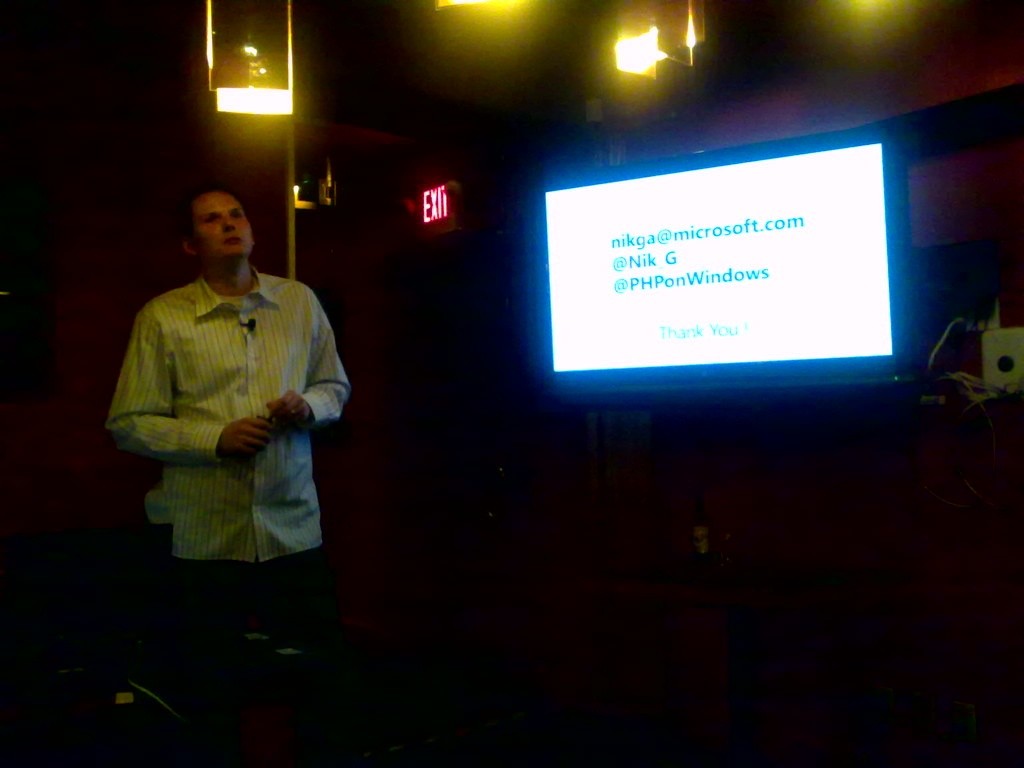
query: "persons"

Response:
[104,181,353,768]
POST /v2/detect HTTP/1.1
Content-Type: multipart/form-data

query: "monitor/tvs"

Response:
[520,132,921,409]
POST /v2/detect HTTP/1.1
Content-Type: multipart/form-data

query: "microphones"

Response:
[246,318,256,331]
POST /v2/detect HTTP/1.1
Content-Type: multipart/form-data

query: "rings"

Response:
[291,409,296,415]
